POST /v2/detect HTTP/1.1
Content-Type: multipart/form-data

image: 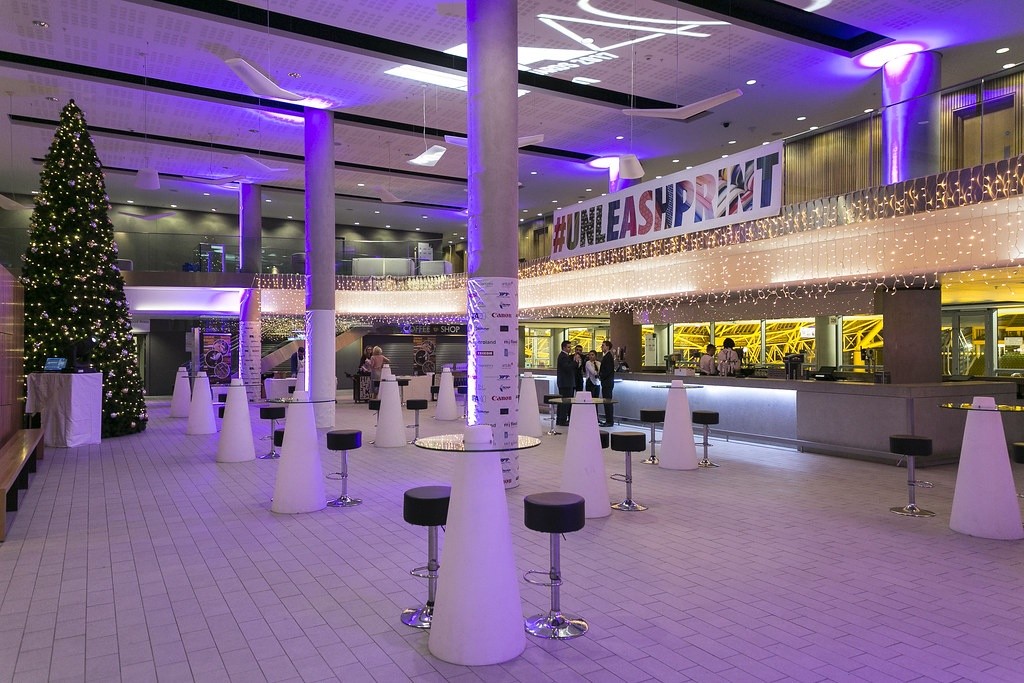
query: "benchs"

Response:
[0,428,46,541]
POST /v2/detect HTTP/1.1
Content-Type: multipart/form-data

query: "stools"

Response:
[523,492,589,640]
[288,386,296,393]
[889,435,936,518]
[218,394,227,403]
[325,430,362,508]
[369,399,381,444]
[600,430,609,448]
[640,409,665,465]
[270,428,284,501]
[692,411,721,468]
[400,486,451,628]
[398,380,408,406]
[458,386,468,419]
[543,394,563,436]
[610,432,648,512]
[430,386,440,419]
[374,381,380,387]
[218,406,225,433]
[1011,442,1024,499]
[258,408,285,459]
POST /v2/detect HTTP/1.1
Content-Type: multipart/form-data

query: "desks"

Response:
[181,372,219,434]
[371,375,412,448]
[939,397,1024,540]
[211,379,263,463]
[415,425,541,666]
[651,380,704,470]
[548,392,619,519]
[518,372,546,438]
[431,367,463,421]
[168,367,197,418]
[374,364,399,399]
[265,391,337,515]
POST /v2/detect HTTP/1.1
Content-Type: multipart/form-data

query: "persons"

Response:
[585,350,602,423]
[556,341,580,426]
[359,346,373,369]
[569,345,586,392]
[291,347,305,378]
[710,338,742,376]
[365,346,391,399]
[699,344,717,376]
[1010,373,1023,377]
[599,341,614,427]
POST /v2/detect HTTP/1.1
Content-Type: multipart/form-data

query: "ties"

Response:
[593,362,599,372]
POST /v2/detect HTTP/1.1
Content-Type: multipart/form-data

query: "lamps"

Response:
[1,0,742,211]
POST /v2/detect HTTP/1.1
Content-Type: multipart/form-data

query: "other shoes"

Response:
[598,421,613,427]
[556,421,569,426]
